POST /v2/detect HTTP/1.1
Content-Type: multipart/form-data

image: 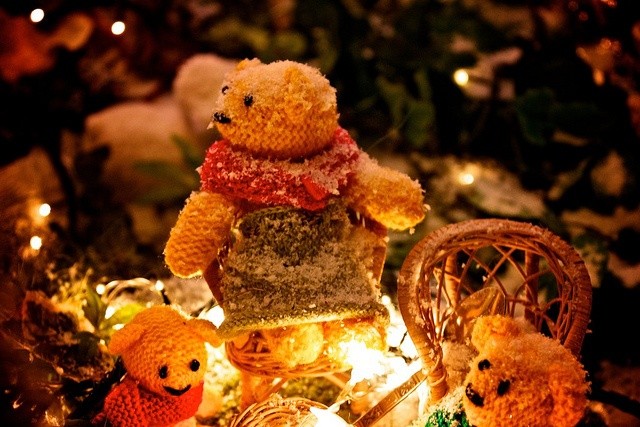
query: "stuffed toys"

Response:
[162,58,428,279]
[91,307,222,427]
[416,315,592,427]
